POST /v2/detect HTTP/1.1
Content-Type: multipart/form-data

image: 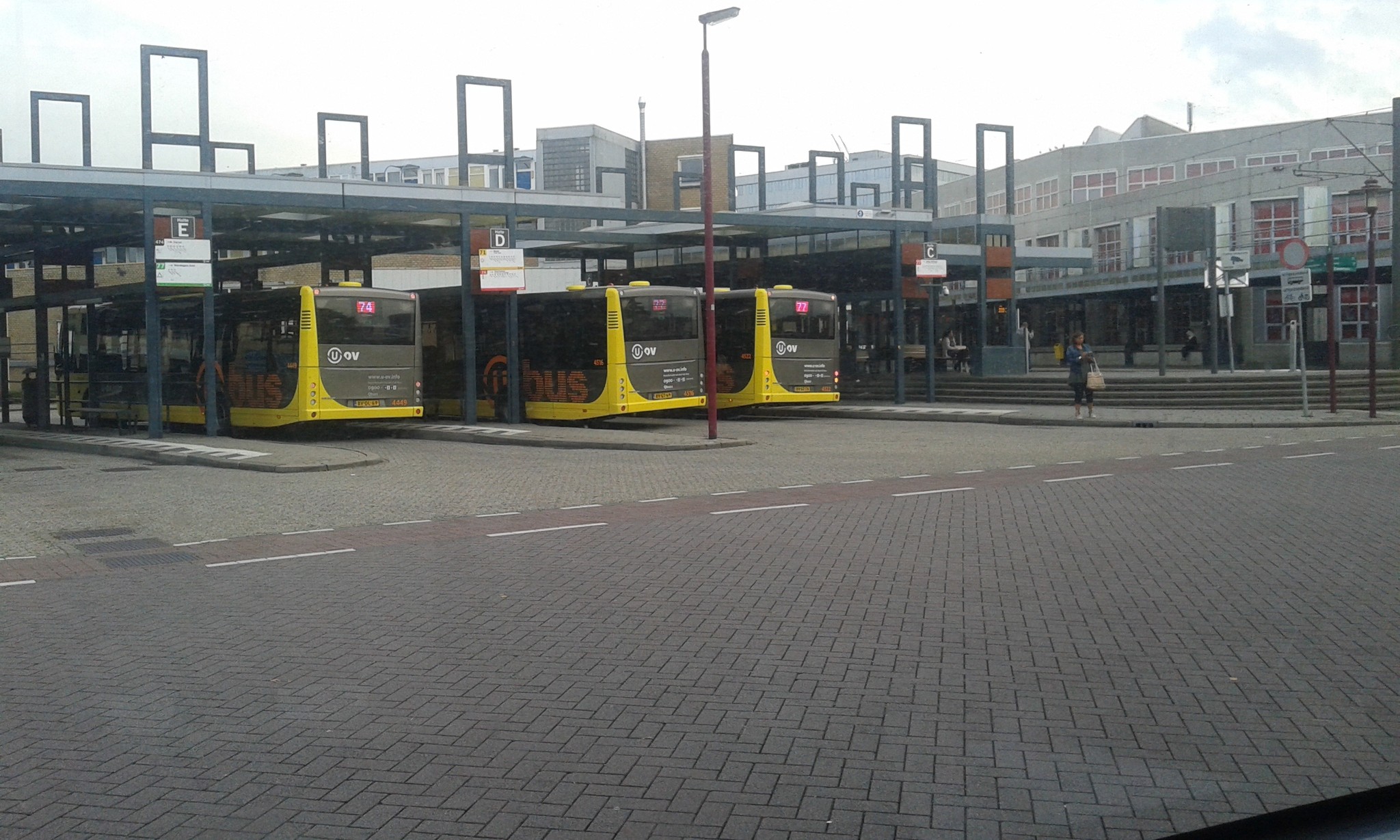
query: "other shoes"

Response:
[1087,413,1094,418]
[1075,413,1082,421]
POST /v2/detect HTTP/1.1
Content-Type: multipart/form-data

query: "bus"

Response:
[52,281,424,441]
[695,283,841,411]
[402,280,709,427]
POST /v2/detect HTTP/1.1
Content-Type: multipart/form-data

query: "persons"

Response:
[1180,330,1198,362]
[934,329,966,370]
[1020,322,1035,372]
[1066,331,1096,419]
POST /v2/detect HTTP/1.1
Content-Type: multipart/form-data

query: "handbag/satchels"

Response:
[1087,355,1105,390]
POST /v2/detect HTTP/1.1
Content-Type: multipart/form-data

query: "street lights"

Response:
[698,5,742,440]
[1360,176,1382,419]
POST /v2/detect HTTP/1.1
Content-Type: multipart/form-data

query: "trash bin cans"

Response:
[20,366,38,429]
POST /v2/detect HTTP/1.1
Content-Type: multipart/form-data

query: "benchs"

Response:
[902,343,971,372]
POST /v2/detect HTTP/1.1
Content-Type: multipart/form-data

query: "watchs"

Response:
[1078,356,1083,362]
